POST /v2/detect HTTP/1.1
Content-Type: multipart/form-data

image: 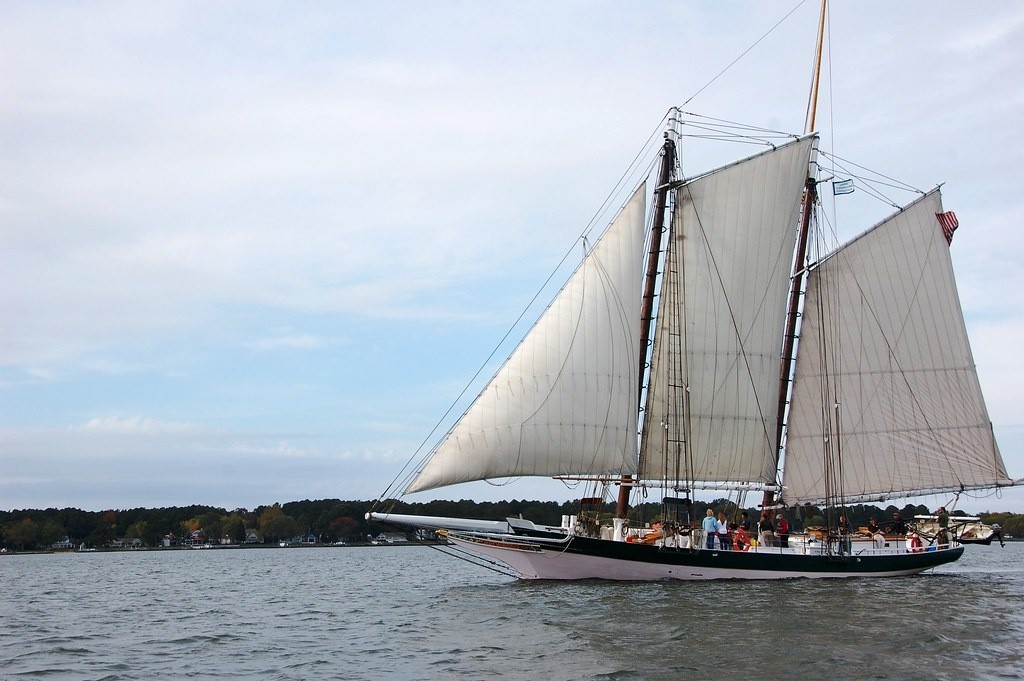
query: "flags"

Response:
[833,178,855,195]
[935,210,959,246]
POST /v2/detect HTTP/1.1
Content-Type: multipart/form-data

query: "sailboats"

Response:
[364,0,1024,580]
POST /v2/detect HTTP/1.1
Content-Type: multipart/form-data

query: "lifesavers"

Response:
[910,538,923,553]
[733,532,751,552]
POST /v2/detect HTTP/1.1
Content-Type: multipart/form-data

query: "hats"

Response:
[742,512,748,518]
[775,514,783,519]
[869,517,876,521]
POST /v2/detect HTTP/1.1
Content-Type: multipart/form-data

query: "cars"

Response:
[1004,533,1012,539]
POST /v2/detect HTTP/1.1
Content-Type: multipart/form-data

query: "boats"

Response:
[912,518,1006,548]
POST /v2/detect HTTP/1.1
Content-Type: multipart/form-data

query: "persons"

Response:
[936,506,949,550]
[867,517,885,549]
[703,508,717,549]
[716,513,728,550]
[837,515,849,535]
[759,513,775,547]
[776,514,790,548]
[888,512,910,553]
[726,512,750,548]
